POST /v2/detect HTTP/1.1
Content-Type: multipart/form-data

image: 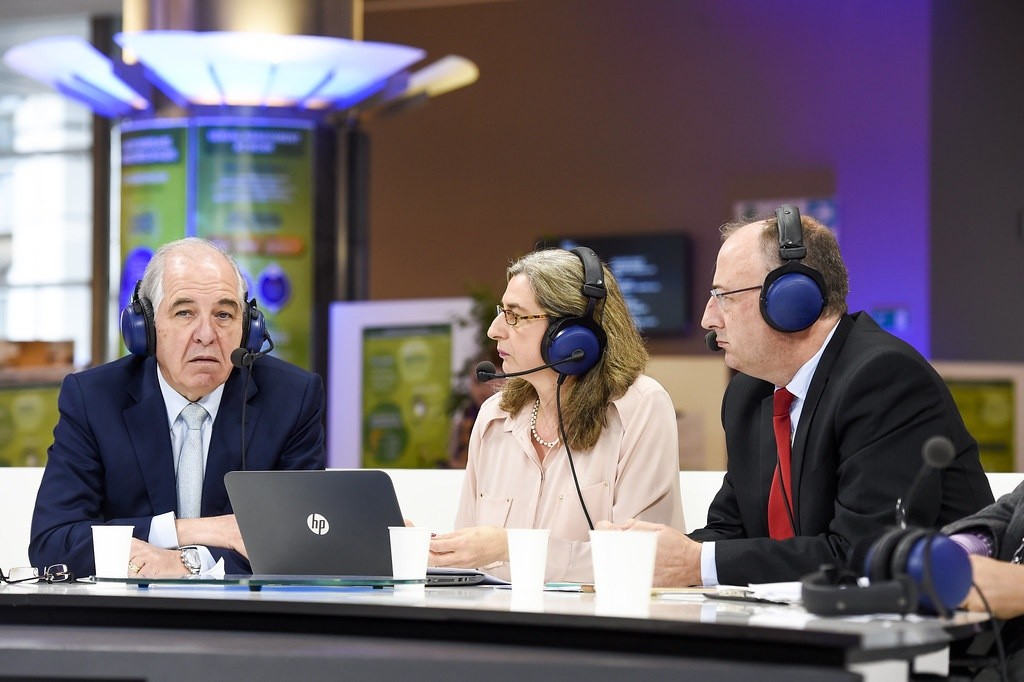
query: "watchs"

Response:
[181,547,201,574]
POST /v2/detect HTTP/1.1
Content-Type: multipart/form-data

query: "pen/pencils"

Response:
[896,498,907,528]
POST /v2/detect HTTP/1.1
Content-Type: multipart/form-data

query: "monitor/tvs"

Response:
[537,231,695,338]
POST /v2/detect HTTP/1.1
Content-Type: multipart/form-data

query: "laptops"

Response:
[223,470,485,587]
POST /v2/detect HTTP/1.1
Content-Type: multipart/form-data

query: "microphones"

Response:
[898,438,956,530]
[230,337,275,368]
[704,331,723,352]
[476,358,573,382]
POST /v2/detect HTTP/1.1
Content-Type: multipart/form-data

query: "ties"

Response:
[766,386,796,542]
[173,406,209,520]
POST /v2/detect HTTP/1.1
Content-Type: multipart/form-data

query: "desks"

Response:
[0,560,998,682]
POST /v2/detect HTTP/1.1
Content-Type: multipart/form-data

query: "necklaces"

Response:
[530,396,555,448]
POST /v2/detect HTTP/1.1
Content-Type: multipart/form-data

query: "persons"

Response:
[938,481,1024,682]
[404,240,687,583]
[619,210,996,659]
[28,233,326,579]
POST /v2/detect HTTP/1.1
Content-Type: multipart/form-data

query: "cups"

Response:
[388,527,433,580]
[589,530,659,599]
[506,529,552,591]
[92,525,135,579]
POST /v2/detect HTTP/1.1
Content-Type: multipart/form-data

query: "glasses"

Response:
[709,285,765,308]
[0,564,76,585]
[494,303,557,326]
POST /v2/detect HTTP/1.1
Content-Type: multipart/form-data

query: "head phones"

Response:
[120,280,271,357]
[760,204,828,333]
[802,524,974,618]
[541,247,607,385]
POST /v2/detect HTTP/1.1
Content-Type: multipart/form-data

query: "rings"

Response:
[128,561,140,573]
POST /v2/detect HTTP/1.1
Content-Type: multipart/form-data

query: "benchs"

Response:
[0,466,1024,585]
